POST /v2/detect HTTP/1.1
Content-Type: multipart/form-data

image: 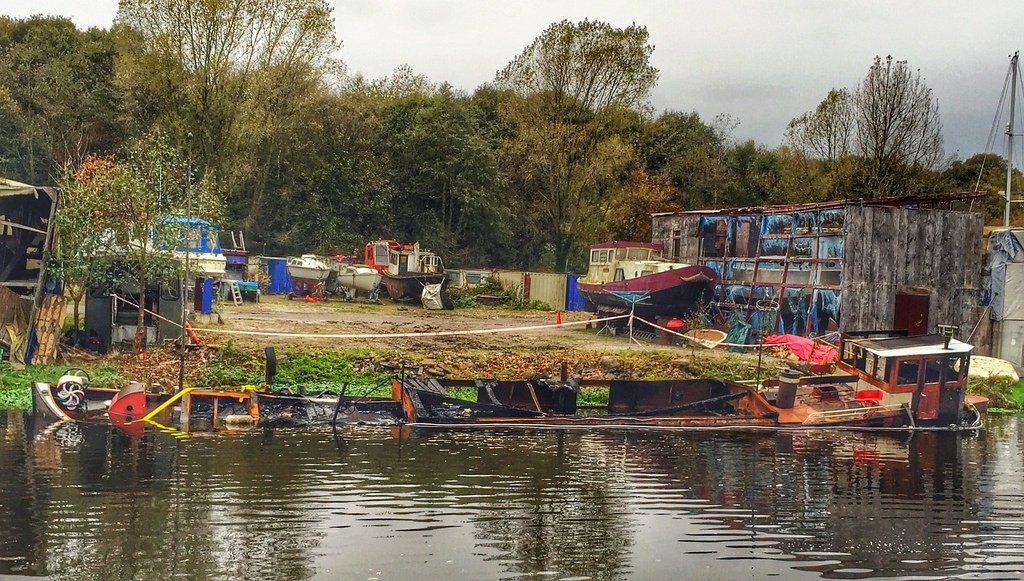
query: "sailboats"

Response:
[74,129,227,279]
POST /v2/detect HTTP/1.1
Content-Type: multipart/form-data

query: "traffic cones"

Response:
[305,295,316,303]
[554,311,563,327]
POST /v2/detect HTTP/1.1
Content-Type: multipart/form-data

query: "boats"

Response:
[394,324,988,437]
[577,241,718,313]
[212,231,259,299]
[337,264,382,290]
[382,243,453,310]
[287,254,330,279]
[32,368,407,433]
